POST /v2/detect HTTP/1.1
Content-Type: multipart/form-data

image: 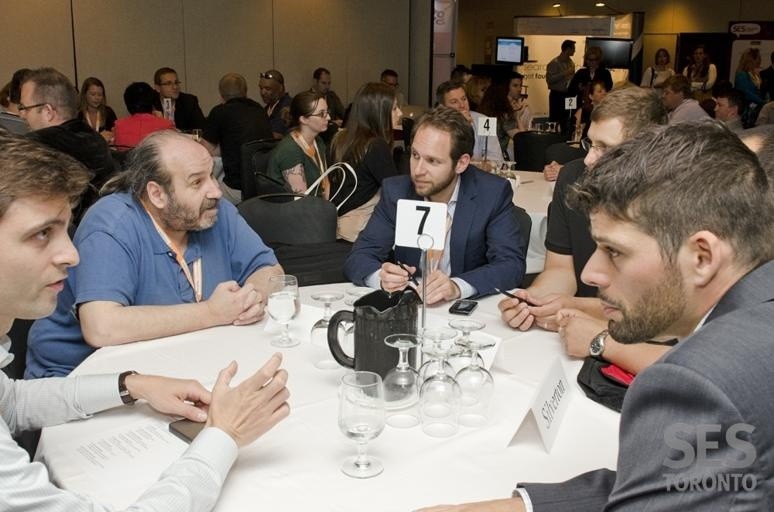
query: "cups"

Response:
[534,124,540,134]
[191,129,202,143]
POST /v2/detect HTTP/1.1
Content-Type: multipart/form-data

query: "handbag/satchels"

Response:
[577,337,678,411]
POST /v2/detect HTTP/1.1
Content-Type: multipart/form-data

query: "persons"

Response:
[0,40,774,512]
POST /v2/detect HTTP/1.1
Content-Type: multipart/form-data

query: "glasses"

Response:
[260,72,281,82]
[311,110,331,117]
[581,137,606,156]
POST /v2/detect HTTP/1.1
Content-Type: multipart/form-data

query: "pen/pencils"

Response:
[494,287,538,306]
[520,179,534,185]
[397,260,418,286]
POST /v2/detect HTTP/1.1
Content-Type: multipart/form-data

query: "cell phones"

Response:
[450,301,478,315]
[169,416,206,443]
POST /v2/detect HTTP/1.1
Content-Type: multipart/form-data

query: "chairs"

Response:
[236,122,343,288]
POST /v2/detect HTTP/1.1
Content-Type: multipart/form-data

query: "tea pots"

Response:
[326,285,424,401]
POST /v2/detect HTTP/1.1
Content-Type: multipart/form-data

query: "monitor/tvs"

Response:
[583,37,633,69]
[496,37,529,65]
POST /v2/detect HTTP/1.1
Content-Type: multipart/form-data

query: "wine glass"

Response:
[382,317,496,438]
[545,122,558,133]
[468,161,517,196]
[266,275,301,348]
[308,287,374,370]
[336,372,388,477]
